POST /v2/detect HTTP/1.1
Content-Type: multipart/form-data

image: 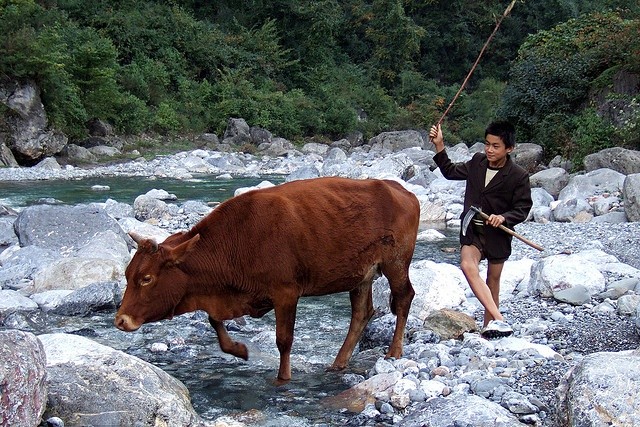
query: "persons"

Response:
[429,121,534,339]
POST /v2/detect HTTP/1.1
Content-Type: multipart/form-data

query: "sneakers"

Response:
[480,319,513,340]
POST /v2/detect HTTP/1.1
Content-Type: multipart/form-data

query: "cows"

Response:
[113,177,420,387]
